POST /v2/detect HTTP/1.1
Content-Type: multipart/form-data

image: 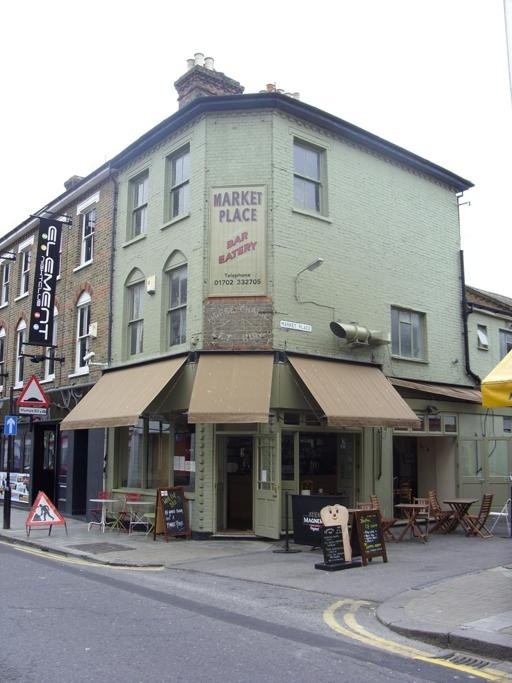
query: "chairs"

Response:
[80,486,158,540]
[354,489,512,544]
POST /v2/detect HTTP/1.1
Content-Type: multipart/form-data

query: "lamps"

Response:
[82,351,108,367]
[292,256,325,283]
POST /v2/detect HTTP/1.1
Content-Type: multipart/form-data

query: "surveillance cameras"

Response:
[83,352,95,361]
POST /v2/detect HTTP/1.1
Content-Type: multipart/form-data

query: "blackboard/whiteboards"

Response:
[355,510,386,558]
[157,487,190,536]
[320,525,345,565]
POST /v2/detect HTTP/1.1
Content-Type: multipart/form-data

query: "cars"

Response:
[281,448,320,476]
